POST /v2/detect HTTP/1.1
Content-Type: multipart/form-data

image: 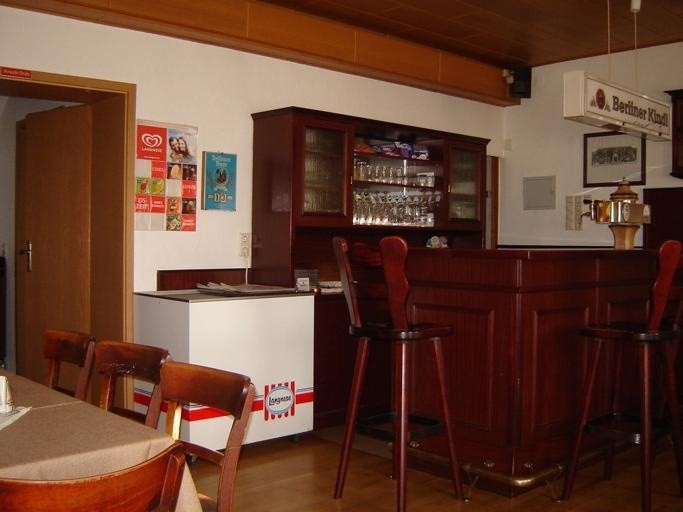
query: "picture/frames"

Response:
[583,131,646,188]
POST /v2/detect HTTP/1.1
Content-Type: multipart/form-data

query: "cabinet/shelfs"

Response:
[333,236,463,512]
[252,106,491,430]
[560,240,683,512]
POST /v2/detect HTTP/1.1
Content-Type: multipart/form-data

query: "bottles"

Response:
[353,153,435,189]
[353,189,440,227]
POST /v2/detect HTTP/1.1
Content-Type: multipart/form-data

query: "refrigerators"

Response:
[132,288,318,464]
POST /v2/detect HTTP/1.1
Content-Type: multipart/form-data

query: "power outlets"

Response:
[239,231,249,257]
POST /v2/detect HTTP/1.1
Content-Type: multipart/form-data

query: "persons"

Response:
[169,136,192,163]
